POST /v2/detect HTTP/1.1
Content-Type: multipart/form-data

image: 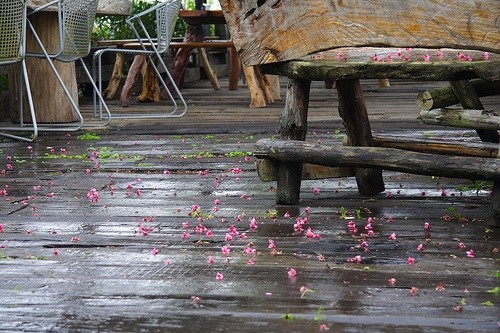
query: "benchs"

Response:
[217,1,500,205]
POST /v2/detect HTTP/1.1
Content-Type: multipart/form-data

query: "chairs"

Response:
[0,0,111,132]
[0,0,39,144]
[92,0,187,119]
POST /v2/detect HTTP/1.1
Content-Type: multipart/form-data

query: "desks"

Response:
[156,9,281,108]
